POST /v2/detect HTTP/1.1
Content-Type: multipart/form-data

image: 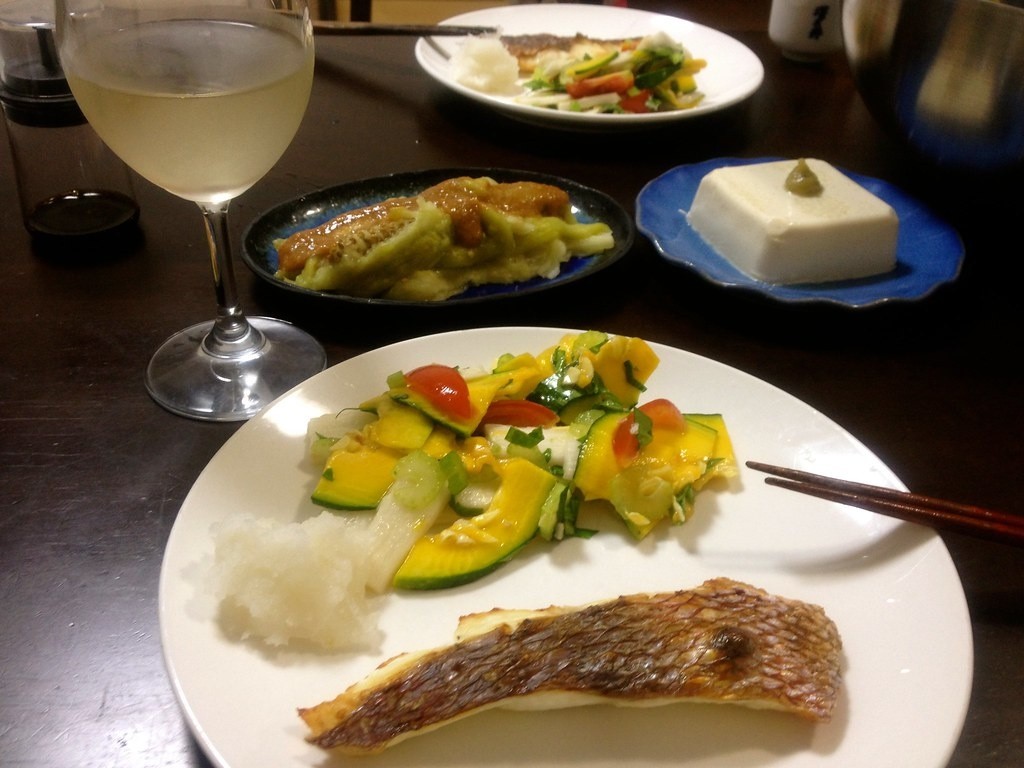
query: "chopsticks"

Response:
[314,18,501,43]
[748,452,1024,550]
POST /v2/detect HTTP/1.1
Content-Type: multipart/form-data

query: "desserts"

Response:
[694,157,903,286]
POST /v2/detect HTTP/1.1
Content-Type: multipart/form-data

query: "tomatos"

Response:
[566,70,658,112]
[401,364,684,460]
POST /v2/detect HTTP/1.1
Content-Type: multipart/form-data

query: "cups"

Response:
[0,4,144,256]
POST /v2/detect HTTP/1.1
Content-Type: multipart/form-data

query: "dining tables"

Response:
[1,23,1024,768]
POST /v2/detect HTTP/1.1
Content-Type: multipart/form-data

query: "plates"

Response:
[636,155,965,313]
[239,166,634,329]
[155,325,977,768]
[408,5,766,123]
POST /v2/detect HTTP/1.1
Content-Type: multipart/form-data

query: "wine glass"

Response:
[50,0,328,425]
